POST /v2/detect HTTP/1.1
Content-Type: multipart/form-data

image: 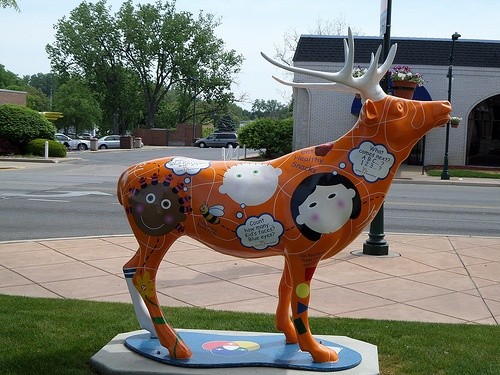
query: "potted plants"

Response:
[119,134,133,149]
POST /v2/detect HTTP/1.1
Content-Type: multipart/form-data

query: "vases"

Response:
[451,121,459,128]
[393,81,417,100]
[356,93,361,98]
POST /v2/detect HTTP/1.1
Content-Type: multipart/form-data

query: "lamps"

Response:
[476,106,489,120]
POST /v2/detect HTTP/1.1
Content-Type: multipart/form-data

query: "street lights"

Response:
[192,75,200,144]
[439,32,462,180]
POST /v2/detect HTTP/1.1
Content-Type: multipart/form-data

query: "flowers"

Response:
[391,66,425,88]
[353,68,368,78]
[451,117,462,123]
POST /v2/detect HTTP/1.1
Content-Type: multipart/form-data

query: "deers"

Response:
[116,26,451,362]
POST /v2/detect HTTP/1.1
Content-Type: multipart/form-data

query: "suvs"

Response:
[195,132,239,149]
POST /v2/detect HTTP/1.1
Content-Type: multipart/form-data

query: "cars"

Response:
[54,132,121,152]
